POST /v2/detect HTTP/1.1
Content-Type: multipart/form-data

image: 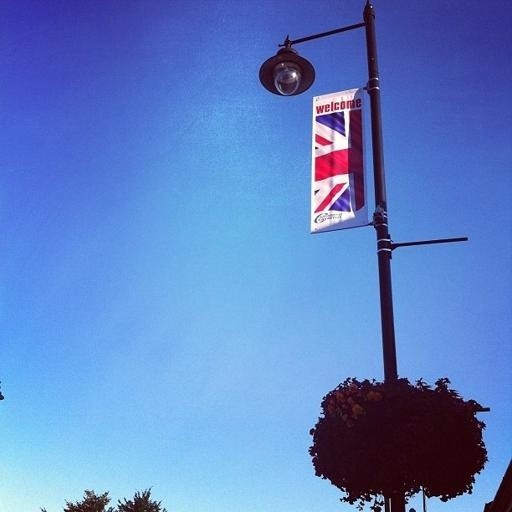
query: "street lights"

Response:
[258,0,407,511]
